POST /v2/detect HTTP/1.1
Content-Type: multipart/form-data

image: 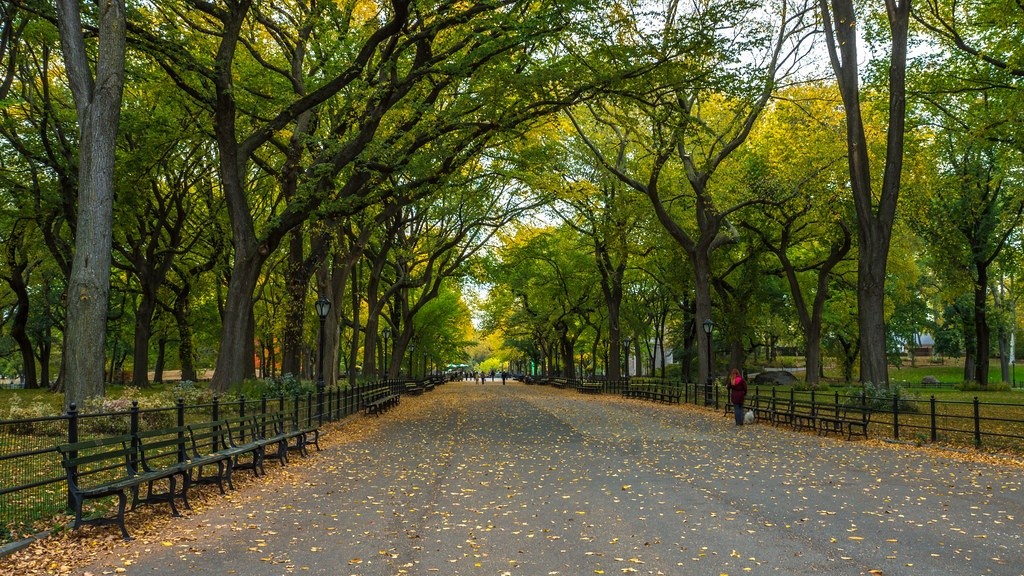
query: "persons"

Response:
[450,370,511,385]
[481,371,486,384]
[726,368,747,425]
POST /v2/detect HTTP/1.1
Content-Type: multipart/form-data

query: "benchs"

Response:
[622,383,683,406]
[187,420,262,491]
[551,379,567,389]
[576,382,603,395]
[361,386,400,417]
[276,410,321,455]
[433,377,440,386]
[723,393,871,441]
[224,416,285,475]
[424,379,435,392]
[537,378,549,386]
[254,412,305,464]
[56,435,182,541]
[404,382,423,396]
[134,426,226,510]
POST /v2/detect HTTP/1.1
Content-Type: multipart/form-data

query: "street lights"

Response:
[703,317,715,404]
[541,358,544,378]
[382,326,391,384]
[579,349,585,392]
[314,294,331,428]
[622,338,631,396]
[423,350,427,377]
[430,354,434,375]
[408,344,414,378]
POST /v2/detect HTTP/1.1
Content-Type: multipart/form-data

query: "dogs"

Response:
[744,409,754,425]
